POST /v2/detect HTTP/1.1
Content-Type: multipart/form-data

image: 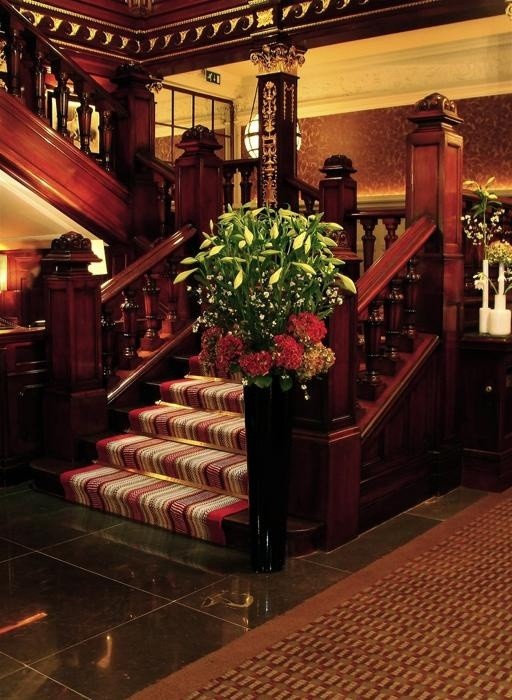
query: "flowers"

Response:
[173,181,358,394]
[457,172,512,294]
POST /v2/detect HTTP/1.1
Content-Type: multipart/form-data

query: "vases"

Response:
[478,259,511,336]
[244,386,294,571]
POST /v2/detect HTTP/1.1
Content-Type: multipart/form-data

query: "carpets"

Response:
[59,356,250,547]
[127,487,511,700]
